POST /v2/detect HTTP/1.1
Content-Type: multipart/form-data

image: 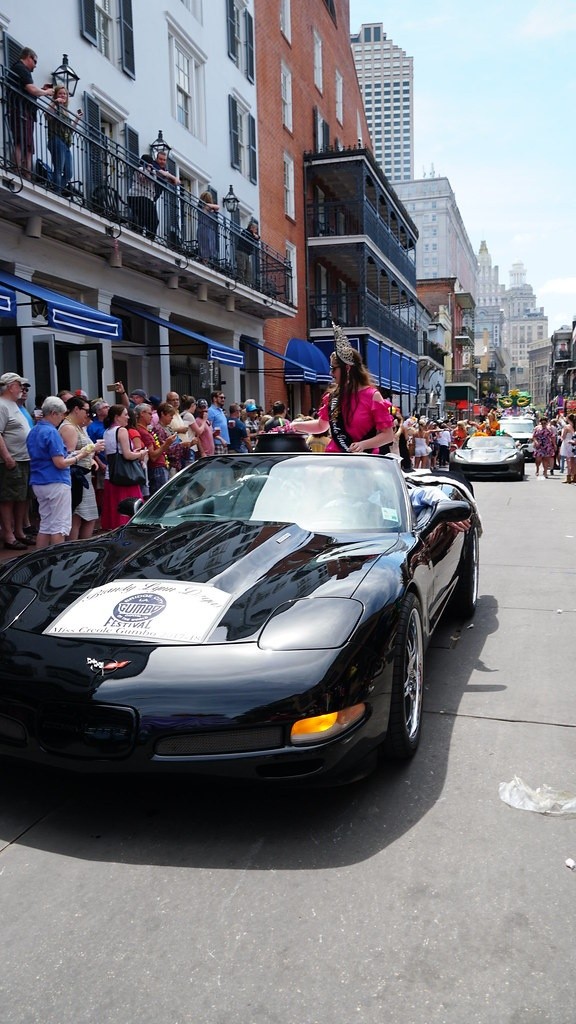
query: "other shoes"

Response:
[15,536,36,545]
[4,539,28,549]
[23,525,38,535]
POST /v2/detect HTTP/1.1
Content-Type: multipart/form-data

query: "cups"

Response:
[34,410,42,416]
[229,420,235,427]
[215,427,220,435]
[97,440,105,444]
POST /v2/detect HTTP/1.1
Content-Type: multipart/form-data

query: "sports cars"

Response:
[0,433,483,792]
[449,432,529,482]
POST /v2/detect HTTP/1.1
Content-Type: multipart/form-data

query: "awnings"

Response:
[0,268,123,341]
[239,338,332,383]
[112,302,247,368]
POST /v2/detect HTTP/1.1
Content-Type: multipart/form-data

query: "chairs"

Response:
[210,256,230,273]
[36,158,85,208]
[171,230,198,257]
[94,185,135,228]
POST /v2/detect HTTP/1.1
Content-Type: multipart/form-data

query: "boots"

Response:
[570,474,576,483]
[563,475,571,484]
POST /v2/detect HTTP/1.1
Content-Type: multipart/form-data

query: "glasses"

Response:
[219,397,226,400]
[330,365,341,372]
[22,388,28,391]
[99,406,109,411]
[74,404,89,414]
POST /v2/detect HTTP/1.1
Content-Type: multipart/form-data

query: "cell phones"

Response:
[49,84,53,96]
[107,384,119,391]
[77,108,82,116]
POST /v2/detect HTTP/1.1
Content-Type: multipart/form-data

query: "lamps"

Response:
[224,185,240,213]
[53,54,80,97]
[150,130,172,162]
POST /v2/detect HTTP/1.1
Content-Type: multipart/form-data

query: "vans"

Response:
[498,416,537,463]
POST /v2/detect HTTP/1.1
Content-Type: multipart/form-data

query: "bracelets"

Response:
[120,390,125,394]
[75,456,78,465]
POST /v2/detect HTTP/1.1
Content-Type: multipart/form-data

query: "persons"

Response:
[317,459,471,532]
[389,407,514,469]
[289,321,394,454]
[44,86,84,196]
[6,47,55,180]
[533,413,576,484]
[127,151,181,240]
[197,191,220,266]
[236,222,261,287]
[0,372,332,549]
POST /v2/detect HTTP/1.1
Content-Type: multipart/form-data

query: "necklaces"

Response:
[65,416,91,470]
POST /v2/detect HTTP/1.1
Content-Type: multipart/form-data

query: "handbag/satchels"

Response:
[67,467,83,513]
[107,426,146,485]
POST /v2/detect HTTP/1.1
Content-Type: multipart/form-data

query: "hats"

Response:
[131,389,151,403]
[75,390,87,397]
[20,377,31,387]
[246,404,257,412]
[150,395,161,406]
[0,372,28,386]
[197,399,208,406]
